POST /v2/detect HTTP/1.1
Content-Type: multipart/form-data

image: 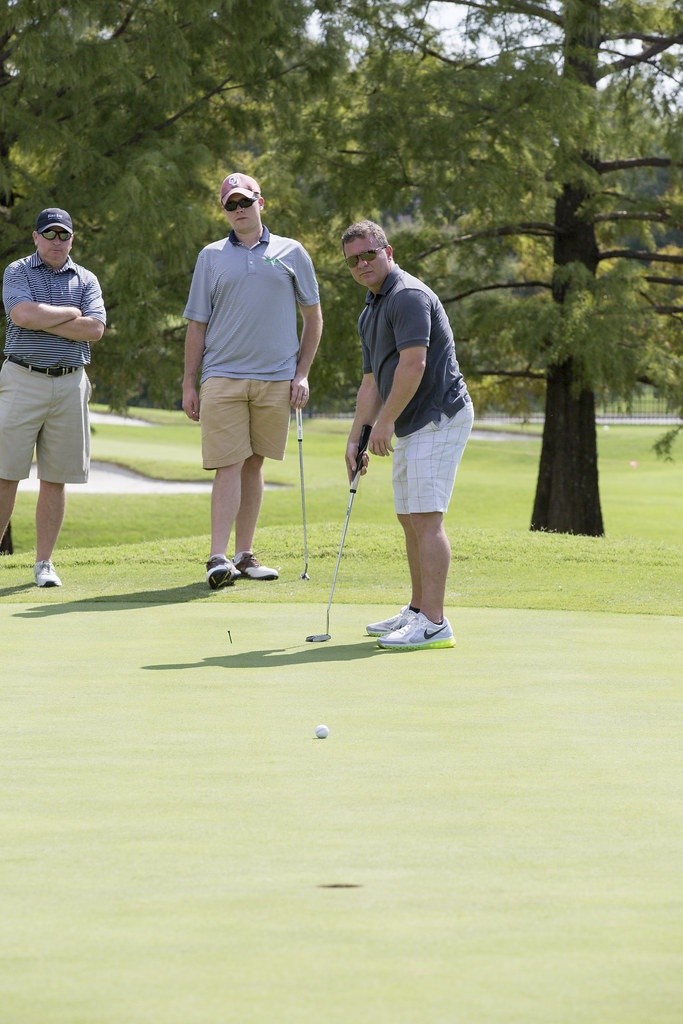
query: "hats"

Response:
[220,173,261,206]
[37,208,74,234]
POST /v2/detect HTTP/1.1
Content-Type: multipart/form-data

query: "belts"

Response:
[9,356,78,376]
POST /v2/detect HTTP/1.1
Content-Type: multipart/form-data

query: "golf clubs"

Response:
[295,408,311,580]
[305,424,372,643]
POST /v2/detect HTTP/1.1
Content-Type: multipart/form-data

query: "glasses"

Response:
[224,197,255,211]
[42,230,71,241]
[344,247,387,268]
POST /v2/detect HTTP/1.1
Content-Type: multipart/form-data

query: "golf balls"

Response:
[315,725,329,738]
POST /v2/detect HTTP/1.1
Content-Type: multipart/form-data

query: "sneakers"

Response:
[232,548,279,578]
[34,561,62,587]
[377,612,456,651]
[199,557,243,590]
[366,602,418,637]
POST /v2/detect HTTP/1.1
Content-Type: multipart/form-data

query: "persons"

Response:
[181,174,322,591]
[0,206,106,588]
[342,219,474,650]
